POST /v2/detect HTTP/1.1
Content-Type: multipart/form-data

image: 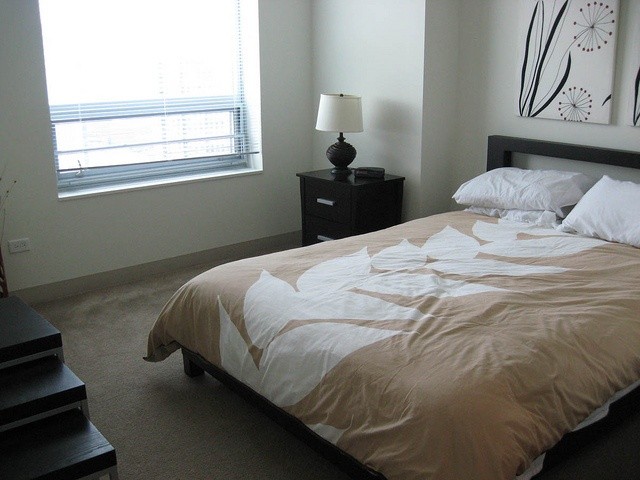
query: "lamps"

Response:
[315,93,364,175]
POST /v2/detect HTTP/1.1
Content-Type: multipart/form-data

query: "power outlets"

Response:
[8,238,31,253]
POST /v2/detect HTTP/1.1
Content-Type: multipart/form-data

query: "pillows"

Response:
[555,174,639,249]
[451,167,597,219]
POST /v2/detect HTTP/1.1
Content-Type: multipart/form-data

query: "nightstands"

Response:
[296,168,405,247]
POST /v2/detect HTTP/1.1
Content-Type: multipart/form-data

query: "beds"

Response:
[140,134,639,480]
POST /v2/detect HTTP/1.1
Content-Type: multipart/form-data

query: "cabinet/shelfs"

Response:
[0,293,118,479]
